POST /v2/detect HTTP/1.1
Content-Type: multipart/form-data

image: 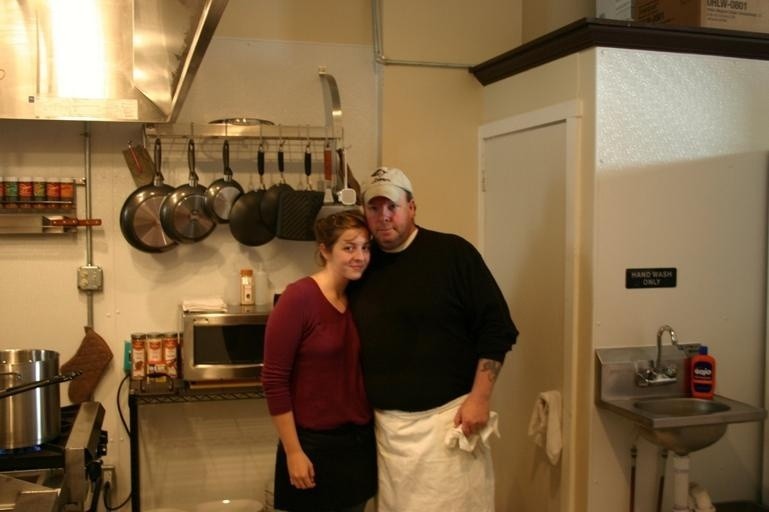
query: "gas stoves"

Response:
[0,401,110,504]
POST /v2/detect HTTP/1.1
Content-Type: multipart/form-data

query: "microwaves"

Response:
[181,305,272,383]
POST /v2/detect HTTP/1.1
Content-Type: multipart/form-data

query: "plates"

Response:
[191,498,263,512]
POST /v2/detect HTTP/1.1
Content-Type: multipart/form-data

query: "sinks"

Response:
[601,393,766,455]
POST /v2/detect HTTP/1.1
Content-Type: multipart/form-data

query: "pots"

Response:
[118,136,336,256]
[0,348,61,449]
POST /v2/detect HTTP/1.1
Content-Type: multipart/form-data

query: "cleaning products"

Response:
[690,345,717,399]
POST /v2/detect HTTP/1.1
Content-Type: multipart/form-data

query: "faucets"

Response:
[634,323,684,388]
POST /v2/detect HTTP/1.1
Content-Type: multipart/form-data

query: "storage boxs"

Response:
[631,0,769,34]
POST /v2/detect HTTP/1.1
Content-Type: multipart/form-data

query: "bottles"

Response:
[0,175,74,209]
[122,331,183,383]
[240,268,257,306]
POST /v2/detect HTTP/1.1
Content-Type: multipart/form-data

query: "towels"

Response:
[444,409,503,460]
[527,386,563,464]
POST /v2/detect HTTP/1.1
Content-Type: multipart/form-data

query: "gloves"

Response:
[59,328,113,403]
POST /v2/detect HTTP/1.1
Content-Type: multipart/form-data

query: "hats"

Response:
[360,165,413,203]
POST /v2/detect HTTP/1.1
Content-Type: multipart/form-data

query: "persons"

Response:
[260,209,381,512]
[337,167,519,512]
